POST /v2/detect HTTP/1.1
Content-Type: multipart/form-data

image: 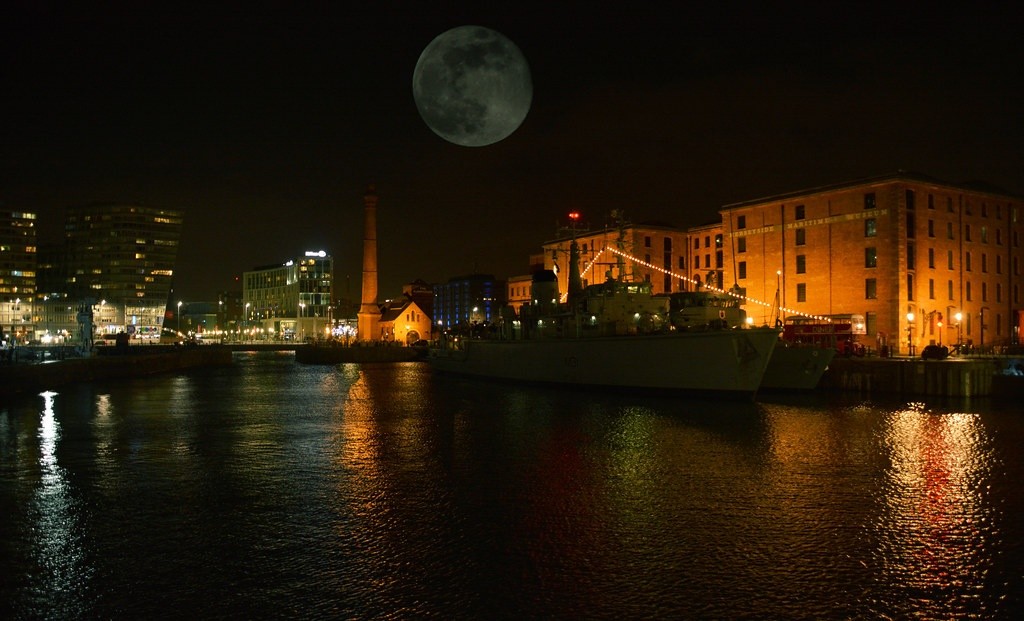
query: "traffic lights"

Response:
[937,312,943,327]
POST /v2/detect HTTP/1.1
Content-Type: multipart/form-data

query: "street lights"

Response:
[956,313,962,354]
[245,303,250,343]
[177,301,182,346]
[101,299,105,333]
[300,303,306,339]
[908,313,913,356]
[15,298,19,341]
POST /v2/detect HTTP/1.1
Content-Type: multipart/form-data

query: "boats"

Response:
[425,209,837,404]
